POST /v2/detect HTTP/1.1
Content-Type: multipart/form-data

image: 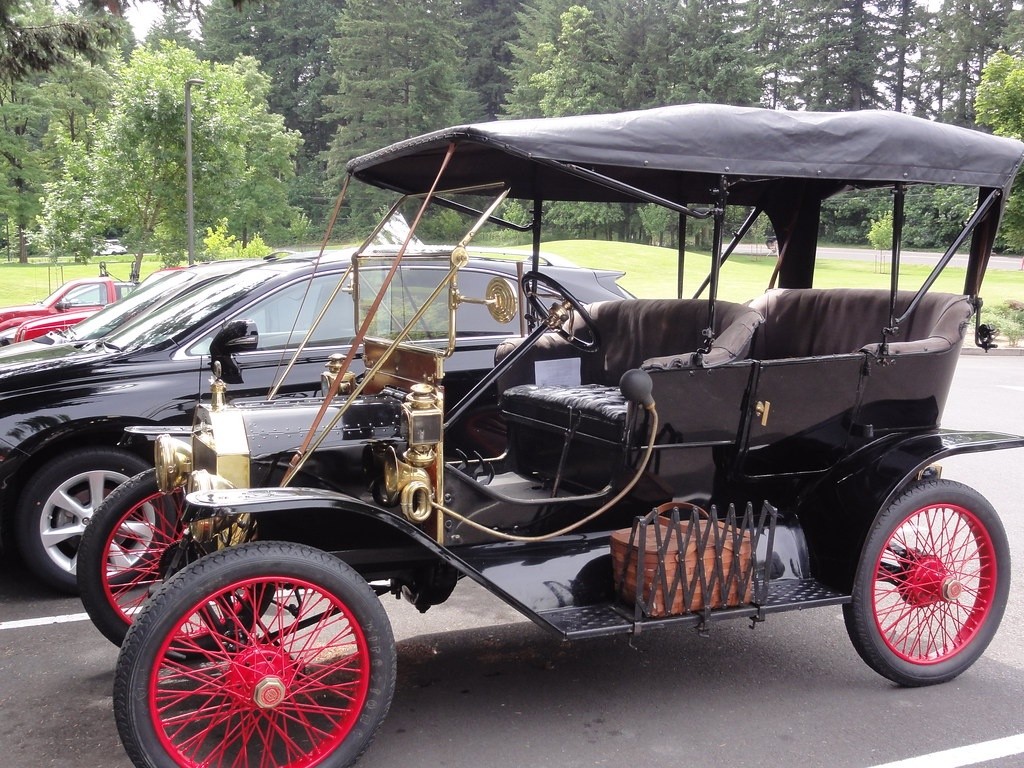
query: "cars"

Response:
[0,276,135,348]
[93,243,127,256]
[78,102,1024,767]
[0,258,311,371]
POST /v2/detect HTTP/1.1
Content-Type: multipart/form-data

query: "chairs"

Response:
[307,274,354,341]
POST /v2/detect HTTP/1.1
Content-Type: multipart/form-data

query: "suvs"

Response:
[0,241,633,597]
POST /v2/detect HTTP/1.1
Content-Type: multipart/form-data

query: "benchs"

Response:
[493,299,766,449]
[747,287,974,357]
